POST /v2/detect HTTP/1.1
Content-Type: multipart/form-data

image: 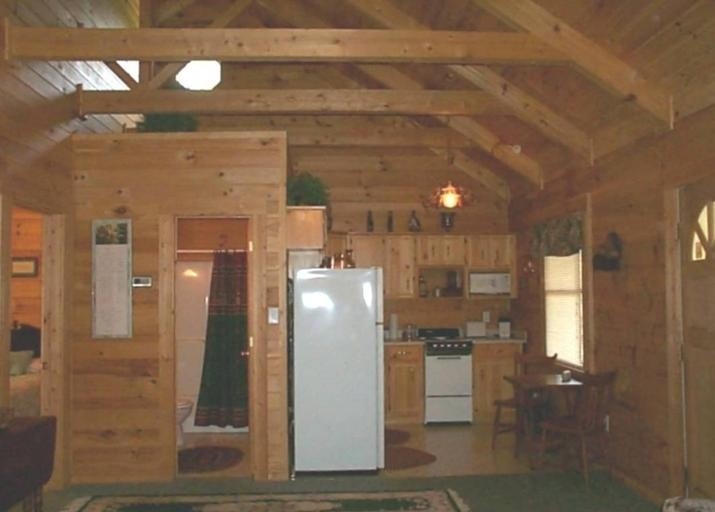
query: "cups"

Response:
[562,371,572,383]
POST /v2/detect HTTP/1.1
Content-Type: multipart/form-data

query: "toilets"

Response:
[176,399,196,450]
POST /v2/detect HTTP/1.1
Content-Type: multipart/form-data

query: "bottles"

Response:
[367,210,373,232]
[408,210,420,232]
[387,210,393,232]
[418,276,429,296]
[384,313,418,340]
[327,249,355,268]
[433,286,442,297]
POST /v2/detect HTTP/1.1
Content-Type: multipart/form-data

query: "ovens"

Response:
[425,354,474,424]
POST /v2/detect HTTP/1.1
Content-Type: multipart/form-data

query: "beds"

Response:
[10,319,46,419]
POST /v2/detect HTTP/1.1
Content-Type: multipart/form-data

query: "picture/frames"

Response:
[90,216,134,340]
[12,256,38,278]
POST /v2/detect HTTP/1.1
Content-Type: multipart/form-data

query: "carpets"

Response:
[176,441,245,475]
[8,474,572,510]
[63,483,472,512]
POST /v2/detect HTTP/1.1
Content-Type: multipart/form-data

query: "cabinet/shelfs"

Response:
[282,202,529,429]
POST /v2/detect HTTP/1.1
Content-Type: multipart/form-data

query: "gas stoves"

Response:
[421,337,471,353]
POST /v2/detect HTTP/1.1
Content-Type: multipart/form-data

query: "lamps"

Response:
[427,132,467,210]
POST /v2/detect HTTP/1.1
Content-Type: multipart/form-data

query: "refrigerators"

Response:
[291,266,385,473]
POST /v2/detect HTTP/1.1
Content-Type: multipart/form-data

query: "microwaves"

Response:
[468,273,510,295]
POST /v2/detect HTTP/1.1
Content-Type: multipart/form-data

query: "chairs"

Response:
[490,349,629,490]
